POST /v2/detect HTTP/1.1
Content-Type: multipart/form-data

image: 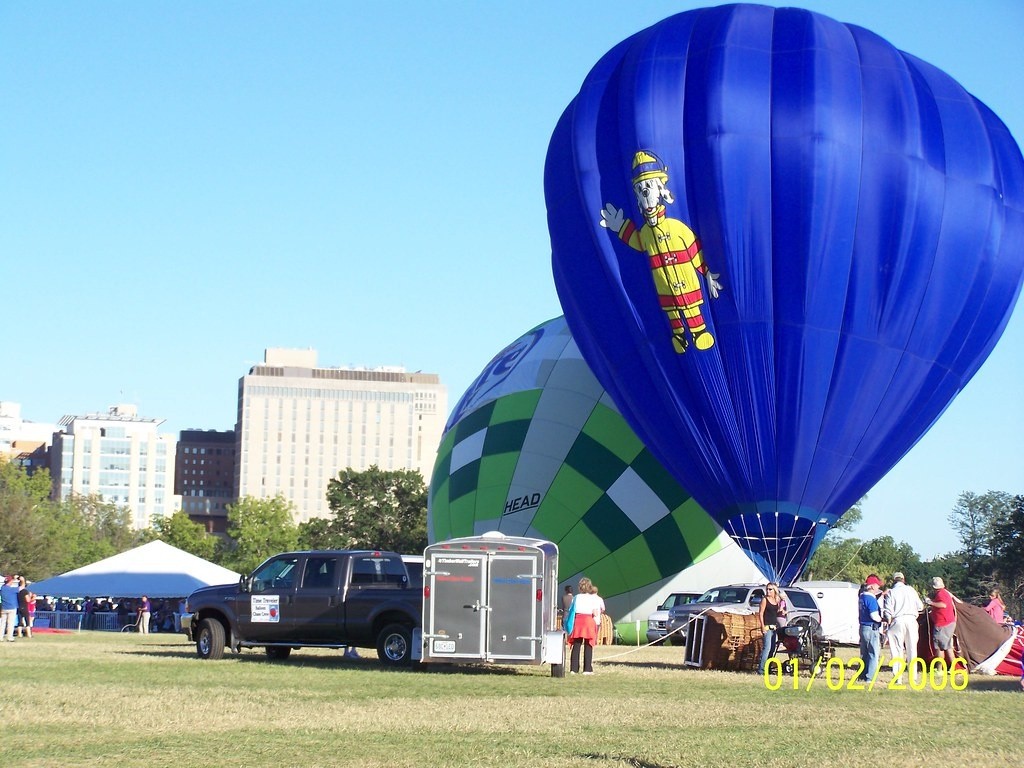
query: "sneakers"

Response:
[927,669,943,676]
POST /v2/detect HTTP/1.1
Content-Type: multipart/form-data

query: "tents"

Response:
[31,538,253,598]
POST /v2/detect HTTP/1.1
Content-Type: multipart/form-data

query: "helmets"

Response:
[932,577,945,589]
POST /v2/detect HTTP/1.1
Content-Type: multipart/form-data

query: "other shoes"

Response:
[343,650,367,661]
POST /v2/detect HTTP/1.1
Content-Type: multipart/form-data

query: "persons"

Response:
[980,587,1012,624]
[563,578,606,676]
[0,575,198,642]
[756,582,787,676]
[857,572,957,684]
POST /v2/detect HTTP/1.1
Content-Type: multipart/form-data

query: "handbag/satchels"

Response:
[564,595,577,635]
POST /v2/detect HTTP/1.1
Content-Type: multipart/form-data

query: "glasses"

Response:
[767,588,773,590]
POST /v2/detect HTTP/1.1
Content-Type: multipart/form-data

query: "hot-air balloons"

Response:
[543,3,1024,590]
[425,312,770,645]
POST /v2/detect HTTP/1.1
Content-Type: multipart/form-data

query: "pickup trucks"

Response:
[179,550,423,665]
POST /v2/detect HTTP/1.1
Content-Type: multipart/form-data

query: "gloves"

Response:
[925,598,932,605]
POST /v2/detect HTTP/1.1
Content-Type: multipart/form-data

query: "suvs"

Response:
[667,584,823,648]
[647,592,705,645]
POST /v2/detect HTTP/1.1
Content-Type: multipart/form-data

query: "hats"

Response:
[868,577,882,586]
[4,576,12,584]
[893,572,904,580]
